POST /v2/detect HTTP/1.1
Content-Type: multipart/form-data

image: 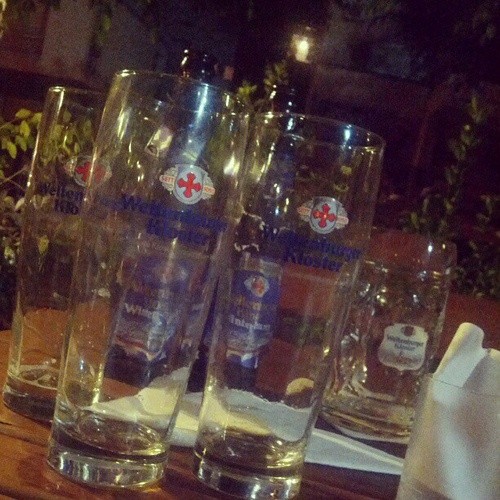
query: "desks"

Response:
[1,261,500,499]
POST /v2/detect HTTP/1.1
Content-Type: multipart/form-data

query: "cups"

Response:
[2,87,171,425]
[190,113,383,499]
[46,68,252,493]
[320,231,457,444]
[395,373,498,500]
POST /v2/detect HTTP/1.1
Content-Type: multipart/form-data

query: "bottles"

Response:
[201,23,322,387]
[112,47,220,392]
